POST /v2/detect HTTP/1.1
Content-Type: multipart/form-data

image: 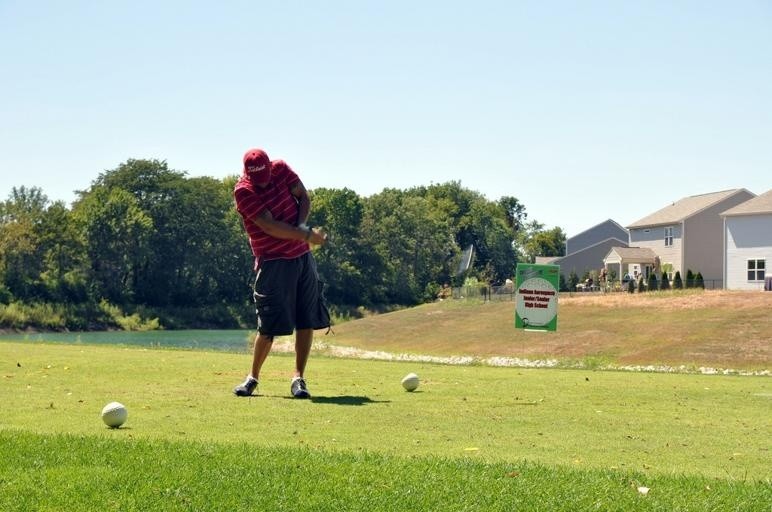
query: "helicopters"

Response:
[101,401,128,427]
[400,373,419,390]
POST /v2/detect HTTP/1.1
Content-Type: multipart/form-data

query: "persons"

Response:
[234,149,332,399]
[598,268,608,297]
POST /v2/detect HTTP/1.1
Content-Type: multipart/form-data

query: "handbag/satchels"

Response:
[304,227,314,244]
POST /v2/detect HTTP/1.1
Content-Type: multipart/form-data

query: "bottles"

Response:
[243,148,273,183]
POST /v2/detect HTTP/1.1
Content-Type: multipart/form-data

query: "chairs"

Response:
[290,376,311,399]
[232,376,260,398]
